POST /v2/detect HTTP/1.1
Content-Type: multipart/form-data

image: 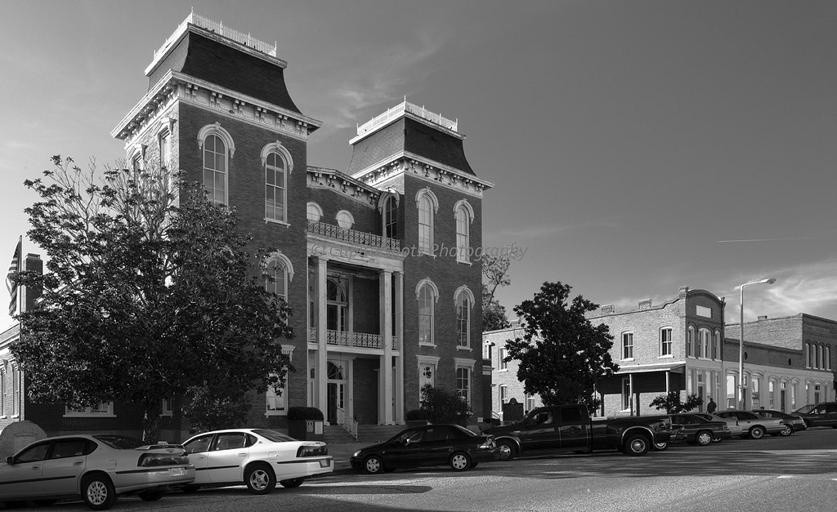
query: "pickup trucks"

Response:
[482,406,675,459]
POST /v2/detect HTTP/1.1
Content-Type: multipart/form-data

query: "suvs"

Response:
[788,401,837,429]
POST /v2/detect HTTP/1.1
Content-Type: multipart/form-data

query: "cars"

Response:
[714,410,787,440]
[175,428,336,495]
[749,410,809,437]
[351,422,497,471]
[651,412,731,450]
[0,435,195,509]
[694,412,743,443]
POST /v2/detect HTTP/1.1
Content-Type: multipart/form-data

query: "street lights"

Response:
[739,278,778,409]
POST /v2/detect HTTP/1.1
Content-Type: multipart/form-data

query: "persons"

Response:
[759,406,766,417]
[707,397,716,415]
[524,410,528,419]
[541,411,553,425]
